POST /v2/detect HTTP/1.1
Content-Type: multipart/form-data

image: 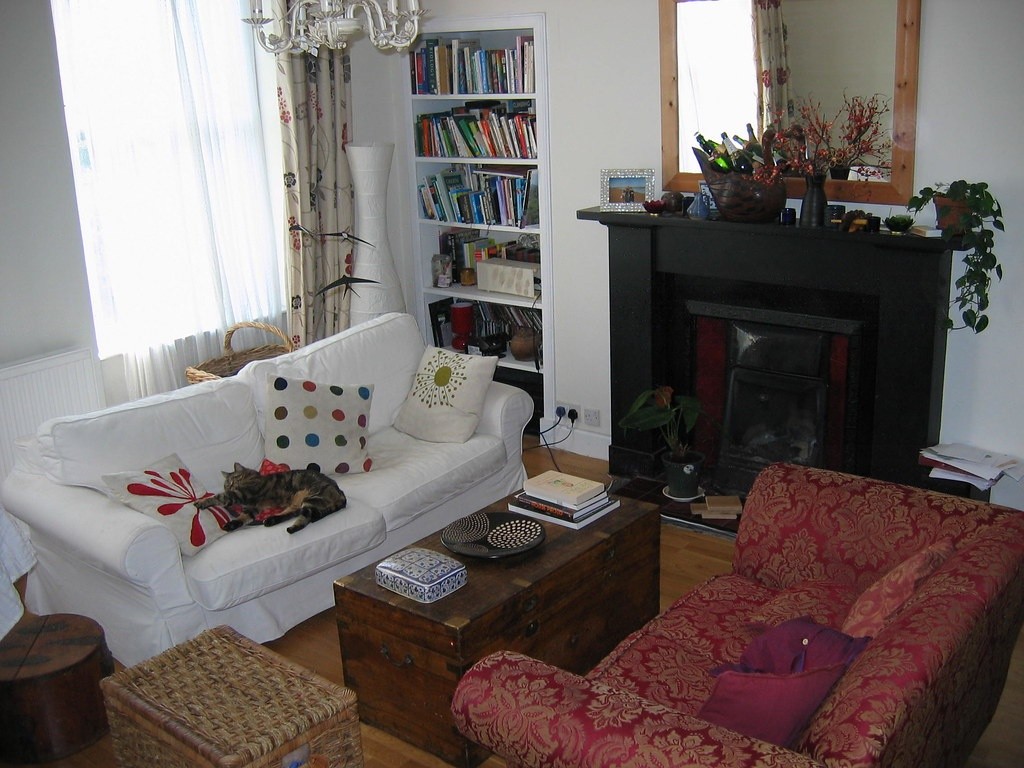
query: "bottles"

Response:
[695,123,788,176]
[779,208,797,225]
[431,254,452,288]
[459,267,475,286]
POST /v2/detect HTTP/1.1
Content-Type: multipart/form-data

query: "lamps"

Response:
[242,0,429,57]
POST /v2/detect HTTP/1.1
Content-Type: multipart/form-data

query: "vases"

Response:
[797,174,828,229]
[830,167,850,181]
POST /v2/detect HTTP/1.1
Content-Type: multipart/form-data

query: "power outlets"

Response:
[556,401,569,426]
[570,404,580,423]
[584,409,599,425]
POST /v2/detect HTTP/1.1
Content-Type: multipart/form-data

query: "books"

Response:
[475,300,541,337]
[418,164,538,229]
[690,495,742,519]
[410,36,535,95]
[507,470,620,531]
[437,225,541,285]
[415,100,538,159]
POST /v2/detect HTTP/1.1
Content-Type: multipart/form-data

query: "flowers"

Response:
[742,91,850,204]
[793,82,892,180]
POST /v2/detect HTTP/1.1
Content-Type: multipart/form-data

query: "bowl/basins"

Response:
[642,200,667,216]
[882,215,915,235]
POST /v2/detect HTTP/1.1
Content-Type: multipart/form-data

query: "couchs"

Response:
[0,314,530,674]
[451,460,1024,768]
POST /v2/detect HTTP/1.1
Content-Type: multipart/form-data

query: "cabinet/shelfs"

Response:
[410,11,552,437]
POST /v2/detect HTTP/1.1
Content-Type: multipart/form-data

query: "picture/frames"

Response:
[600,169,654,214]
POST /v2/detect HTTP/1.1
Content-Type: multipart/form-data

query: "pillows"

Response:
[103,453,254,555]
[265,375,373,475]
[393,344,498,442]
[740,614,872,671]
[841,537,954,638]
[697,671,843,750]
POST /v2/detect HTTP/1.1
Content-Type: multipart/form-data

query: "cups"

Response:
[682,197,694,217]
[864,216,881,232]
[828,204,845,228]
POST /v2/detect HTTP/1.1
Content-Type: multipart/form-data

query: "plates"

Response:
[441,511,545,559]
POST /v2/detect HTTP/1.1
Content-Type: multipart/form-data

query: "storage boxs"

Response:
[332,490,660,768]
[376,547,467,606]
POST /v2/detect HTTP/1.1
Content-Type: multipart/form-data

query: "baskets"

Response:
[185,322,295,385]
[97,624,364,768]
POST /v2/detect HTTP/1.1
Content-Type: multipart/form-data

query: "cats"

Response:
[193,462,348,534]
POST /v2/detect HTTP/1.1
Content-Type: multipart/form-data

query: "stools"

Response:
[0,614,118,768]
[100,626,362,768]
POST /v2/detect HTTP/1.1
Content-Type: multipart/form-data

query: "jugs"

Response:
[687,193,709,221]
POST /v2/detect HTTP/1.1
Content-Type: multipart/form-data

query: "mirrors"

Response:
[660,0,922,206]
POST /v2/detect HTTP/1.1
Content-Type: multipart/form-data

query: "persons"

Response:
[623,185,634,203]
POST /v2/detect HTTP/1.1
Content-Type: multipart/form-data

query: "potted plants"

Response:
[907,180,1005,336]
[619,386,737,498]
[886,215,915,234]
